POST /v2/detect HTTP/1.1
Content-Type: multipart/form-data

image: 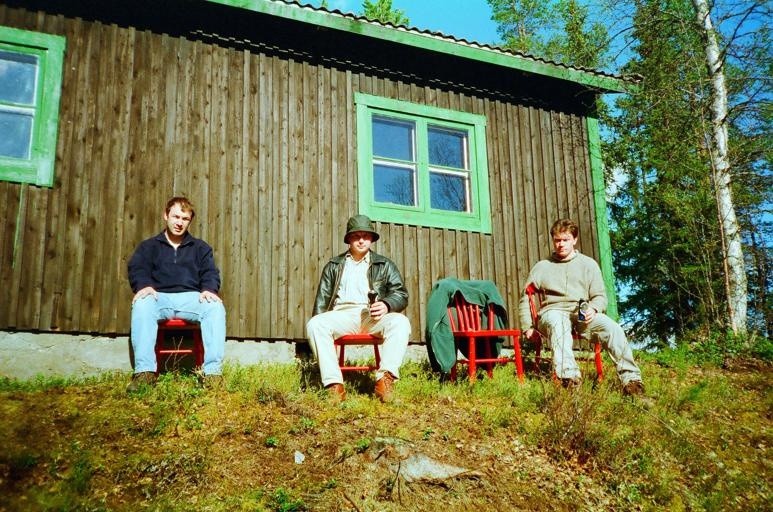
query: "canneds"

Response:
[579,298,589,322]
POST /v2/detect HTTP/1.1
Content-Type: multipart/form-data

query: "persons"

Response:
[127,197,226,393]
[305,215,411,408]
[519,218,646,396]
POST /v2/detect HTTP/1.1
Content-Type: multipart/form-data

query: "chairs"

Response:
[524,282,605,384]
[439,280,525,389]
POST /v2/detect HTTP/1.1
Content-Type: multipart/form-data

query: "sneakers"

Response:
[626,381,646,395]
[126,372,156,392]
[374,371,394,402]
[328,384,346,406]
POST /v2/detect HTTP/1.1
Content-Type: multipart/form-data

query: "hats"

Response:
[344,215,379,243]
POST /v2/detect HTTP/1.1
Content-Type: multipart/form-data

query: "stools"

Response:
[333,334,385,393]
[154,317,203,376]
